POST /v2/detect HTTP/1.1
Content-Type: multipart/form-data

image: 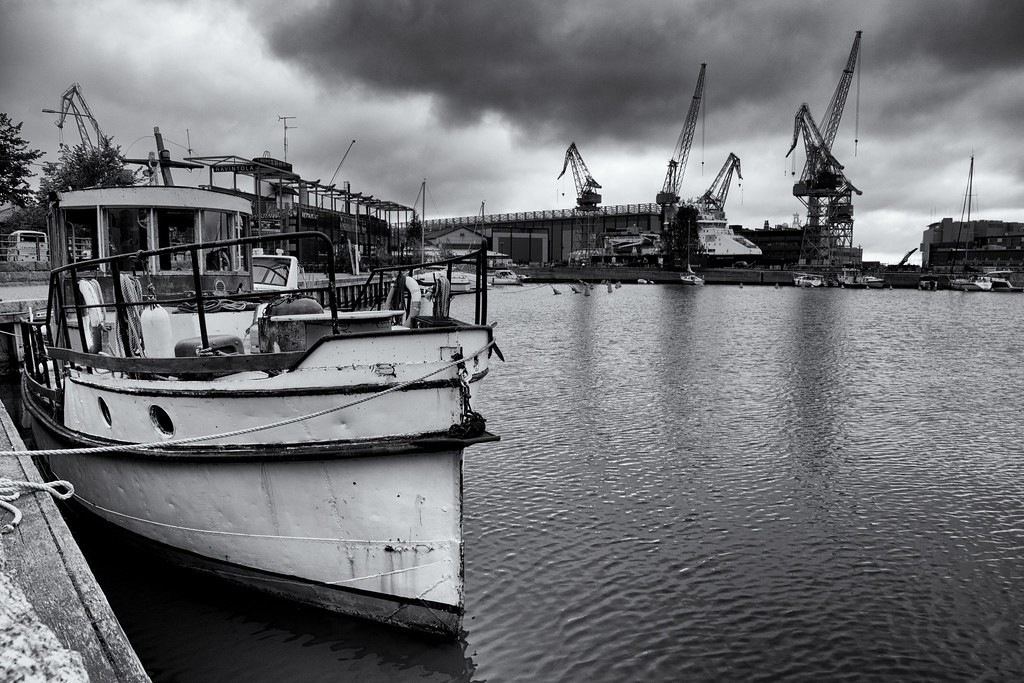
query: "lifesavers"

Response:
[385,276,422,326]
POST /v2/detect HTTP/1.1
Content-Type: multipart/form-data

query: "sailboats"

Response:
[680,217,706,286]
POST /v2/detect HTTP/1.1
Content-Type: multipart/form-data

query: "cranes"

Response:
[691,154,744,223]
[557,142,608,212]
[657,60,709,236]
[784,29,865,268]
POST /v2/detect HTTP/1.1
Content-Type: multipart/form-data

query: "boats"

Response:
[15,171,509,650]
[829,267,869,288]
[481,269,524,286]
[415,270,491,293]
[919,153,1024,293]
[861,276,886,288]
[636,277,656,286]
[792,272,823,286]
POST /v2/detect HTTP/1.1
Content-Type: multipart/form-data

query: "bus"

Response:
[4,230,49,263]
